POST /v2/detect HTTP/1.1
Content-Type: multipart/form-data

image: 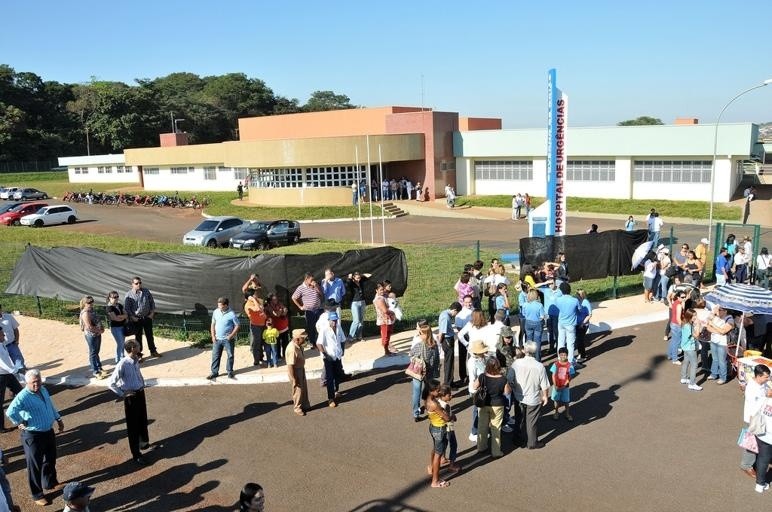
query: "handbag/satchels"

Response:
[123,316,136,336]
[748,405,766,436]
[664,262,682,279]
[765,266,772,277]
[474,373,488,408]
[405,342,427,382]
[513,279,523,292]
[737,427,760,456]
[484,282,497,296]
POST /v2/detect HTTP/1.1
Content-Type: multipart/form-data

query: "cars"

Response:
[749,152,761,162]
[0,201,24,215]
[12,186,49,201]
[0,202,49,226]
[17,204,79,227]
[0,186,20,201]
[227,219,303,252]
[181,215,254,249]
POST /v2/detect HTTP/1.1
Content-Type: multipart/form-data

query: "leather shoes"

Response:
[740,465,756,481]
[141,443,160,450]
[415,414,428,423]
[136,455,151,465]
[34,484,66,506]
[227,372,234,378]
[206,374,219,379]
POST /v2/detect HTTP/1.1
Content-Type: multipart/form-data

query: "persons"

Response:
[350,175,458,209]
[750,185,759,199]
[87,188,94,205]
[244,175,248,191]
[744,185,755,201]
[511,194,519,221]
[236,180,243,201]
[516,193,523,219]
[524,193,531,220]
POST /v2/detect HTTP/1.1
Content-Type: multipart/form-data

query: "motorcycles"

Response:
[59,188,209,210]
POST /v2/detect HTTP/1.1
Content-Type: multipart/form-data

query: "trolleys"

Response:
[726,341,746,371]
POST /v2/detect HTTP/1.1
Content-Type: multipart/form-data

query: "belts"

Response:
[429,421,447,429]
[5,340,16,347]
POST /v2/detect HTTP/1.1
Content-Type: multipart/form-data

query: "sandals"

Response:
[427,463,432,475]
[446,466,462,474]
[439,459,450,468]
[430,478,451,488]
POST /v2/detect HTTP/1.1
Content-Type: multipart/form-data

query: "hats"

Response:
[328,310,340,321]
[659,248,670,254]
[700,238,711,245]
[292,328,306,339]
[468,340,490,355]
[62,480,96,501]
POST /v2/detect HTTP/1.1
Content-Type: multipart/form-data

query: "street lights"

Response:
[707,77,772,252]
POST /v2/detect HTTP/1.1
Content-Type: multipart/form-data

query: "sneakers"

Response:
[92,368,111,380]
[151,350,162,357]
[469,415,515,442]
[136,352,144,363]
[295,392,339,416]
[529,440,545,449]
[663,336,726,390]
[564,413,573,422]
[754,482,770,493]
[553,411,560,420]
